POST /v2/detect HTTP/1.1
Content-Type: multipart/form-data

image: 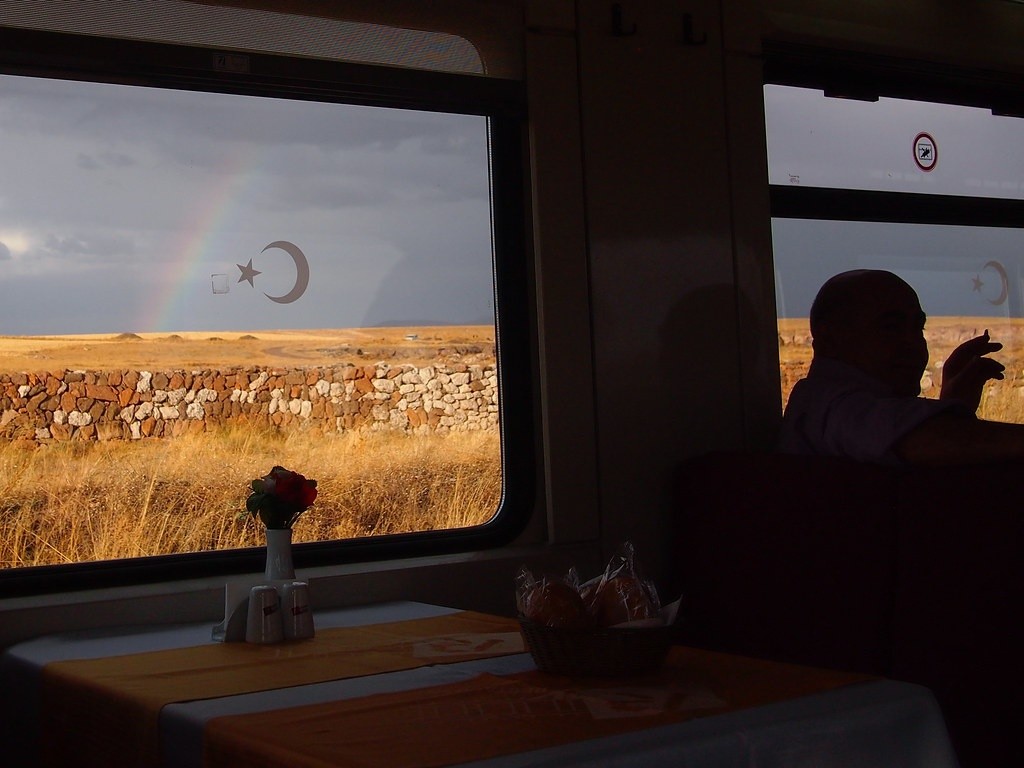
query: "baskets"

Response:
[517,613,677,678]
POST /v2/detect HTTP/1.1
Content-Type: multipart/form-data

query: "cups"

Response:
[280,581,316,642]
[246,585,282,643]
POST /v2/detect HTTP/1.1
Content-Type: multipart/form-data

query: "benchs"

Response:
[672,451,1024,768]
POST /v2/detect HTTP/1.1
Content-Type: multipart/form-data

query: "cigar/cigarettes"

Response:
[977,329,988,356]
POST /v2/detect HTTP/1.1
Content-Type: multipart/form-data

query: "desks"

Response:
[0,595,959,768]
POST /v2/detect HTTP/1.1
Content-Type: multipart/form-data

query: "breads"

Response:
[580,572,660,628]
[525,581,596,628]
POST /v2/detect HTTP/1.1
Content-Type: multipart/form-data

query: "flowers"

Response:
[248,465,319,528]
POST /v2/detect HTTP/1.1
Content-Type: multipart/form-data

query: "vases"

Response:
[264,528,296,586]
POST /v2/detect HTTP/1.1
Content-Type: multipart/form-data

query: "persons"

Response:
[779,270,1024,462]
